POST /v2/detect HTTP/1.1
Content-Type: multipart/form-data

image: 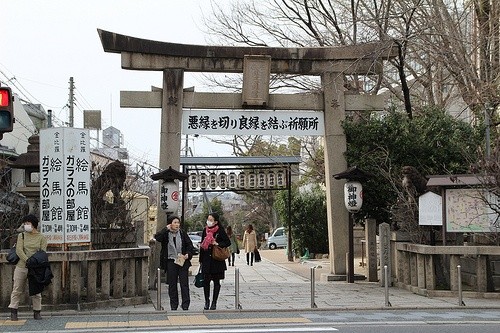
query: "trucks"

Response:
[267,226,288,250]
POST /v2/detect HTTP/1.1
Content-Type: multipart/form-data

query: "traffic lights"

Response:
[0,86,15,141]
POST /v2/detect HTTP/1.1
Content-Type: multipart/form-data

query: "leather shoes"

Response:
[210,301,216,310]
[204,300,210,310]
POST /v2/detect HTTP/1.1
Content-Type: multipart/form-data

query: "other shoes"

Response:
[171,307,177,310]
[183,307,188,310]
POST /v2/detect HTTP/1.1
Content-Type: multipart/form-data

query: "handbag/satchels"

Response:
[237,249,239,254]
[6,232,24,265]
[193,265,204,288]
[255,249,261,262]
[212,245,230,260]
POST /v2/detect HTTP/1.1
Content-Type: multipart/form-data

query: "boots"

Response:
[34,310,42,320]
[11,308,18,321]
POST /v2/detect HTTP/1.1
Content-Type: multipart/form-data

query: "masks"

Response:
[24,224,34,232]
[207,220,215,227]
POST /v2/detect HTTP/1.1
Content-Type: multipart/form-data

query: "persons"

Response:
[153,212,257,310]
[8,214,47,321]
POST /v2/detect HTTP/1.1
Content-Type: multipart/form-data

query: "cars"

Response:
[188,231,202,253]
